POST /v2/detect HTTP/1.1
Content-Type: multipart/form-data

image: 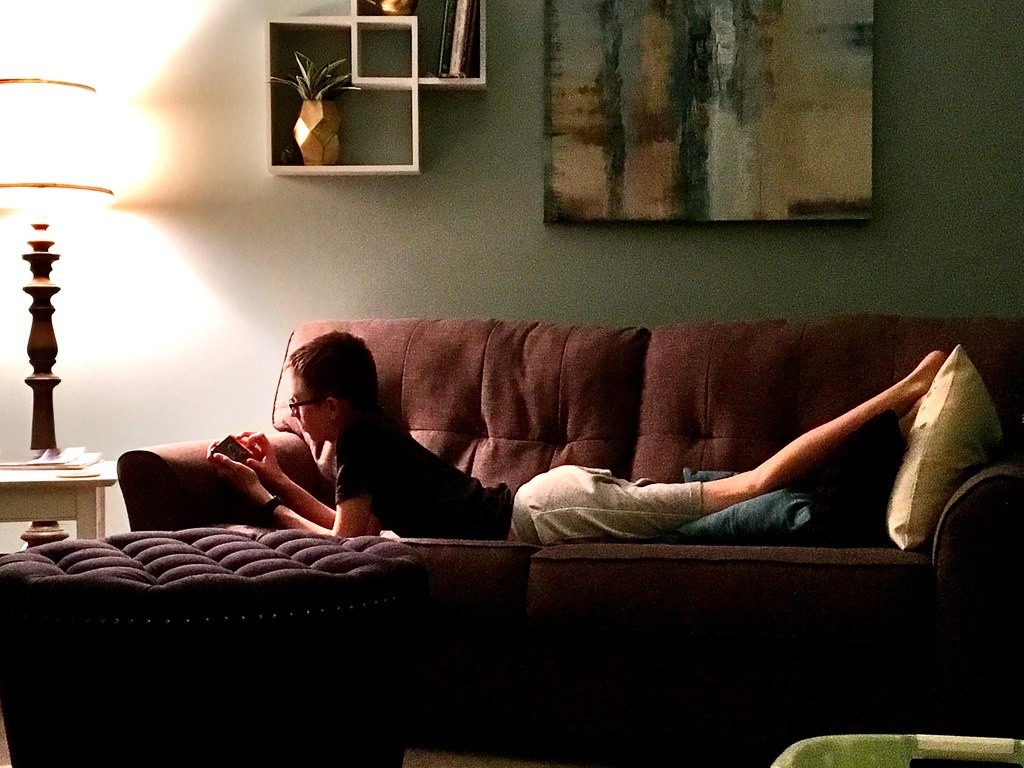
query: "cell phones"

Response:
[213,436,252,464]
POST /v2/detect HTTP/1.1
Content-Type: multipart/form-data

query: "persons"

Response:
[207,331,947,545]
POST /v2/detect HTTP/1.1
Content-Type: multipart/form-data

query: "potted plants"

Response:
[266,51,363,166]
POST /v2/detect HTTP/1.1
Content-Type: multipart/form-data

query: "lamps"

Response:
[0,78,115,450]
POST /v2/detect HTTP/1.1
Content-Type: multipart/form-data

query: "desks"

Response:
[0,459,117,539]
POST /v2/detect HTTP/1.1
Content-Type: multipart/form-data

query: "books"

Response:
[0,447,102,470]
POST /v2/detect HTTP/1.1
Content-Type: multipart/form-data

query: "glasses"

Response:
[288,393,347,418]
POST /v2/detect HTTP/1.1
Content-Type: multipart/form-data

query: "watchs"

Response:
[263,495,284,512]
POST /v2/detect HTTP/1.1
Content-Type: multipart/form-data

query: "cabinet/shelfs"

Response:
[264,0,487,176]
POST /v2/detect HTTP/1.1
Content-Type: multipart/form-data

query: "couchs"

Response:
[114,319,1024,753]
[1,528,428,768]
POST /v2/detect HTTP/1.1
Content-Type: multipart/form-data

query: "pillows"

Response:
[885,343,1004,550]
